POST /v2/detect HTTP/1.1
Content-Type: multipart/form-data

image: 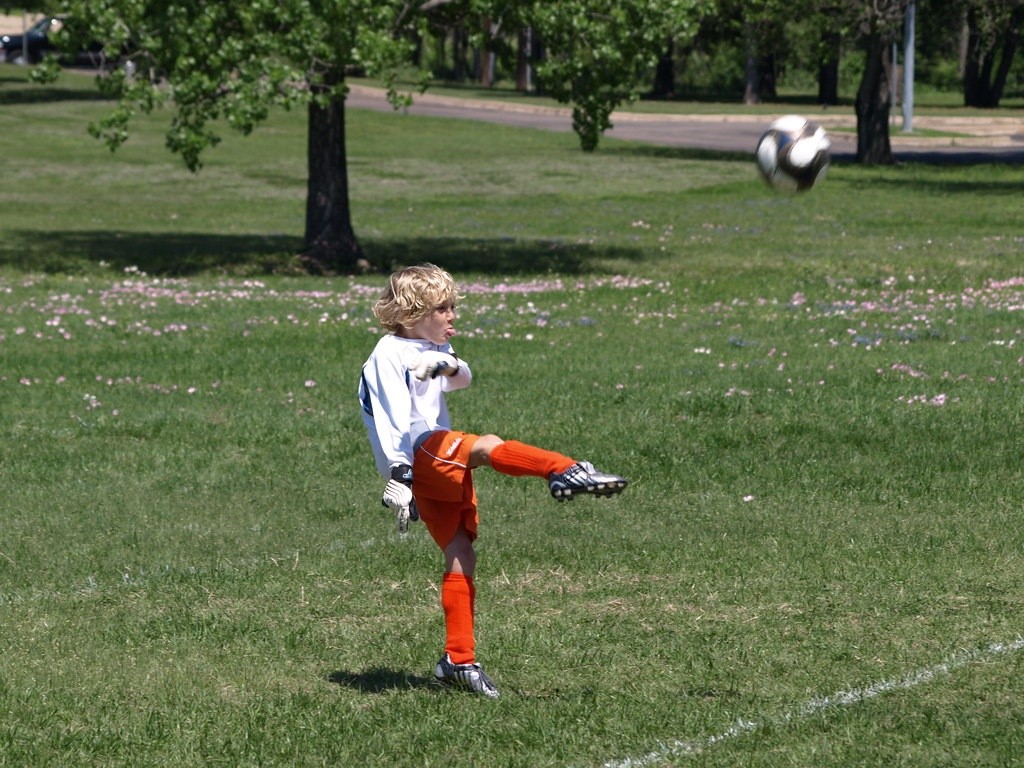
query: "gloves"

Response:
[382,464,419,534]
[407,349,459,382]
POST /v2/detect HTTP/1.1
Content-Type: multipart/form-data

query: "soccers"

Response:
[755,114,830,194]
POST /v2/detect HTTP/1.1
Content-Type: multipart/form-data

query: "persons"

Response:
[356,263,630,697]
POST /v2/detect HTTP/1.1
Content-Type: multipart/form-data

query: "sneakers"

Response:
[549,461,627,502]
[433,652,500,701]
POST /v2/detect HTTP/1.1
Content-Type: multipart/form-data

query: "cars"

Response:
[1,16,162,86]
